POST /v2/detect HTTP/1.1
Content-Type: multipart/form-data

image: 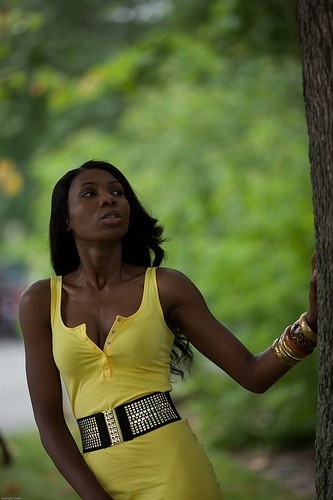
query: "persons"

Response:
[16,160,321,500]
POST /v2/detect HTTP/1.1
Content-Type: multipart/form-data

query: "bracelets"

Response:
[270,312,320,368]
